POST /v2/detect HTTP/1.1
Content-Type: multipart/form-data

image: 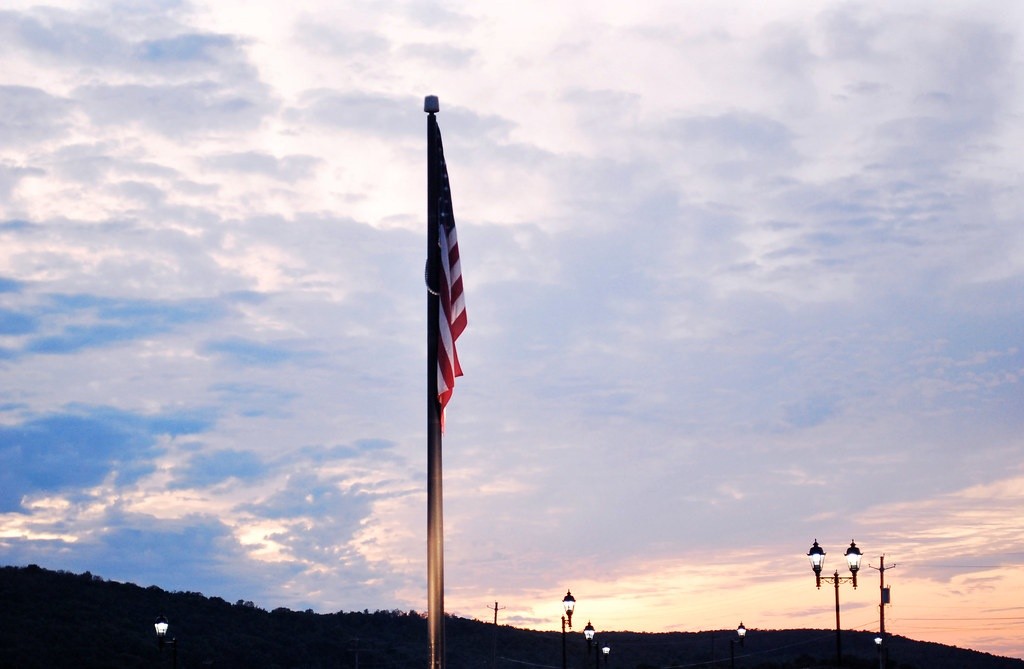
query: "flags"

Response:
[425,114,468,438]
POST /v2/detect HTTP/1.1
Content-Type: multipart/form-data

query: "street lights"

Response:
[806,538,865,669]
[584,620,600,669]
[873,636,882,669]
[602,642,611,668]
[561,589,576,669]
[485,601,507,669]
[154,614,179,669]
[730,621,747,669]
[868,552,897,669]
